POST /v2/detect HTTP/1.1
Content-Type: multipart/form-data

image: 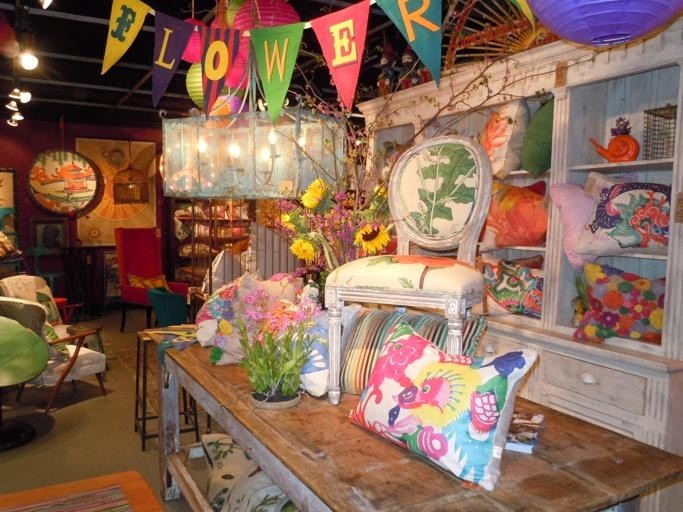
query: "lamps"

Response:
[156,36,347,202]
[112,133,152,205]
[4,0,54,128]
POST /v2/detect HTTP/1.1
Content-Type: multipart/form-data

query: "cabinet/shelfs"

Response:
[349,15,681,512]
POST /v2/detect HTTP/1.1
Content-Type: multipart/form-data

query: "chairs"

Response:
[113,226,189,334]
[0,272,109,453]
[321,133,495,405]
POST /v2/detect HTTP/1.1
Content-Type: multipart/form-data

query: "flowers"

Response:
[264,28,610,311]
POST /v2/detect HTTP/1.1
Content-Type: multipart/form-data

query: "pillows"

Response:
[127,270,174,295]
[197,431,300,511]
[169,196,303,303]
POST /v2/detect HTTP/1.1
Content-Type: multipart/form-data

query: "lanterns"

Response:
[182,2,302,116]
[527,1,683,50]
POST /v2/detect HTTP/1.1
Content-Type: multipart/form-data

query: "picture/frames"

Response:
[30,216,71,258]
[1,166,22,276]
[102,250,121,305]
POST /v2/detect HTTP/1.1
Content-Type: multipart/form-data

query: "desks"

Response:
[144,326,683,511]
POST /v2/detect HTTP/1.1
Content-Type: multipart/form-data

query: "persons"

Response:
[37,225,62,270]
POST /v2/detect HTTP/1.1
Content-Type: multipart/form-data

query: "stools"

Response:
[133,329,200,451]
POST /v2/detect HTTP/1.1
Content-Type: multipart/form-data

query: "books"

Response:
[504,413,546,454]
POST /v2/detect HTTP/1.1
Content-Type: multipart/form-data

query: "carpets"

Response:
[0,467,170,512]
